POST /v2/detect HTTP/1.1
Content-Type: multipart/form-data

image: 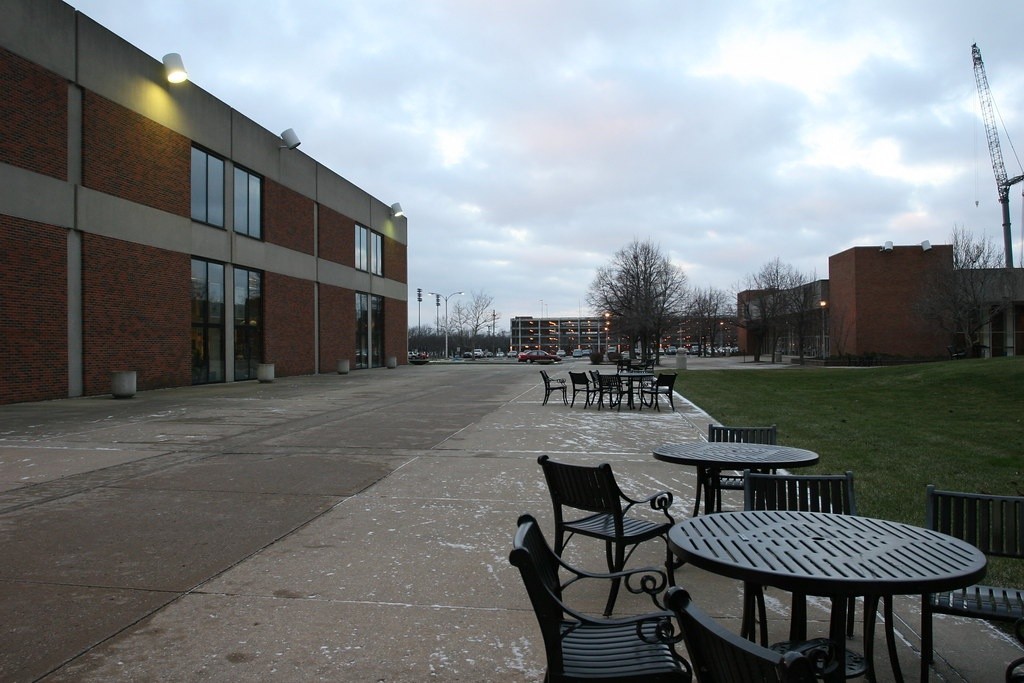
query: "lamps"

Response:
[879,242,893,252]
[279,128,302,150]
[389,203,403,217]
[920,240,932,251]
[162,53,187,83]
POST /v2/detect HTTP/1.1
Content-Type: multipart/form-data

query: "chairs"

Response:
[509,515,694,683]
[707,424,779,513]
[537,455,677,616]
[944,346,966,360]
[743,469,860,643]
[923,484,1024,683]
[540,370,568,406]
[663,587,838,683]
[568,358,678,412]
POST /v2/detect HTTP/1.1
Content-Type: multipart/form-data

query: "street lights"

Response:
[427,291,465,360]
[820,300,826,360]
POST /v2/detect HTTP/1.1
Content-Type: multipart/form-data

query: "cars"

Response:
[496,352,505,357]
[518,349,562,364]
[507,350,518,358]
[573,349,592,357]
[408,350,429,359]
[462,348,494,359]
[607,345,739,363]
[555,349,566,357]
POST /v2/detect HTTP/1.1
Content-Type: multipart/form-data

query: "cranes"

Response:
[971,42,1024,267]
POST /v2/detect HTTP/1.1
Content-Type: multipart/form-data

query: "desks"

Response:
[618,373,654,409]
[667,510,990,683]
[653,442,820,568]
